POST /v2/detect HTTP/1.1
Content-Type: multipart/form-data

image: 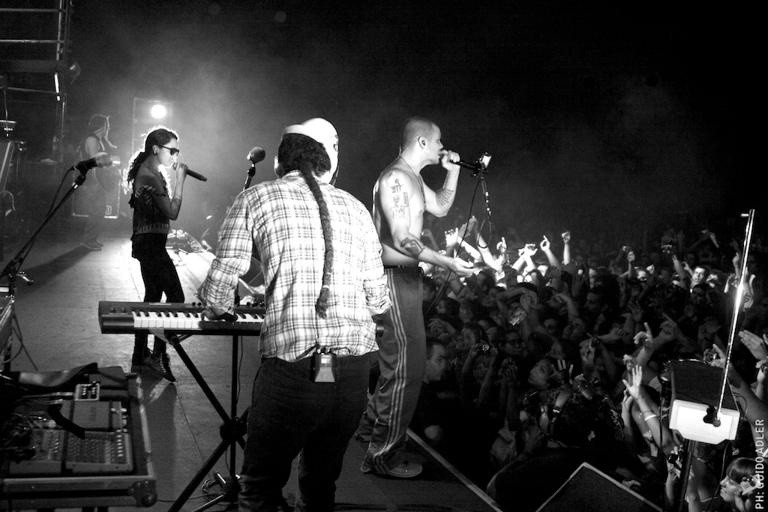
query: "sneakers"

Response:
[131,349,176,382]
[354,429,372,441]
[81,240,103,250]
[375,459,422,477]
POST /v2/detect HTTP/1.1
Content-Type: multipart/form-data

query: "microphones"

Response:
[439,153,479,172]
[172,161,207,182]
[247,147,266,166]
[74,153,113,169]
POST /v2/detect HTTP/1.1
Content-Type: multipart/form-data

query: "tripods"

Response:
[193,173,251,512]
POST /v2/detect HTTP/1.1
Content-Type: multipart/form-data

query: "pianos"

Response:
[99,301,267,336]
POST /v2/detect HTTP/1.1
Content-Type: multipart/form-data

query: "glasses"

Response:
[158,144,179,155]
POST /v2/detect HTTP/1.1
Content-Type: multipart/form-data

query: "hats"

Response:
[275,118,338,183]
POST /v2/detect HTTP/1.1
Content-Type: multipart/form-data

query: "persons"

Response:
[124,125,187,385]
[201,213,218,253]
[370,217,767,509]
[356,115,476,477]
[197,115,396,512]
[79,111,116,252]
